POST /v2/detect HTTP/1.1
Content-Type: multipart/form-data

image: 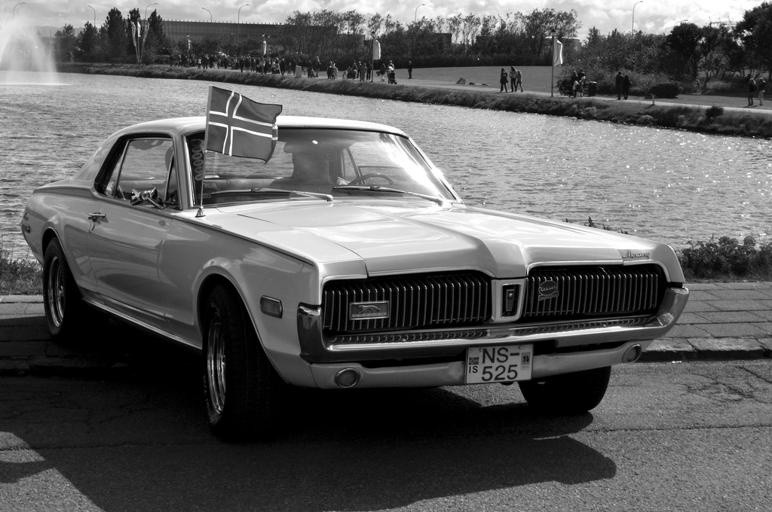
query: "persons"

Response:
[622,75,633,100]
[173,51,338,81]
[614,71,624,101]
[509,66,517,93]
[498,68,507,93]
[746,75,756,106]
[154,142,218,204]
[757,79,766,106]
[408,60,413,79]
[570,68,586,98]
[271,140,341,195]
[515,70,523,92]
[343,59,399,85]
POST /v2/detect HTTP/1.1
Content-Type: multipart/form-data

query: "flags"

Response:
[262,41,267,56]
[371,39,382,61]
[203,86,283,167]
[552,39,563,67]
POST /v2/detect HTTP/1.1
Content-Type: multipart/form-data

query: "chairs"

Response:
[120,142,338,204]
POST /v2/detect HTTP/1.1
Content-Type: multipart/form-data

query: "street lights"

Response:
[414,2,427,21]
[371,30,376,82]
[85,3,100,30]
[11,1,25,25]
[143,1,159,24]
[235,2,252,42]
[260,32,268,58]
[550,27,559,98]
[185,31,191,52]
[629,0,645,36]
[202,6,214,24]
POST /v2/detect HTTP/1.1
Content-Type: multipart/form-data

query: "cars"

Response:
[18,115,691,443]
